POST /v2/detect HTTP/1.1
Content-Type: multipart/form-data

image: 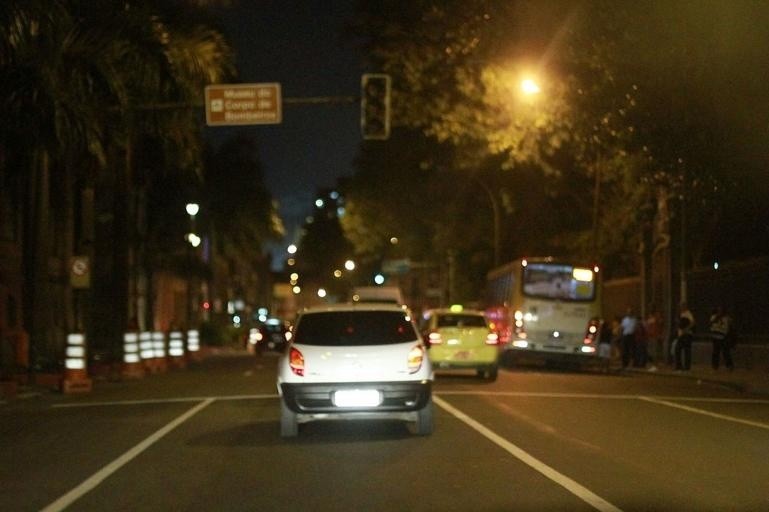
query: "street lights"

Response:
[183,197,201,331]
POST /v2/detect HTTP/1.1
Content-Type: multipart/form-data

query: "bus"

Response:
[476,254,607,372]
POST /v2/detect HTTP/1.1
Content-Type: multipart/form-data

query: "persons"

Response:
[707,300,735,375]
[593,298,698,376]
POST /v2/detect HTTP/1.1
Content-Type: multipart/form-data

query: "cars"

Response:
[248,278,500,384]
[270,305,435,439]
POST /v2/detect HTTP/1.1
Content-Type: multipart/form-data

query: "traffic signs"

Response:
[203,79,281,127]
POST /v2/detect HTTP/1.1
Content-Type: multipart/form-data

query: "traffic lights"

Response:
[362,73,393,140]
